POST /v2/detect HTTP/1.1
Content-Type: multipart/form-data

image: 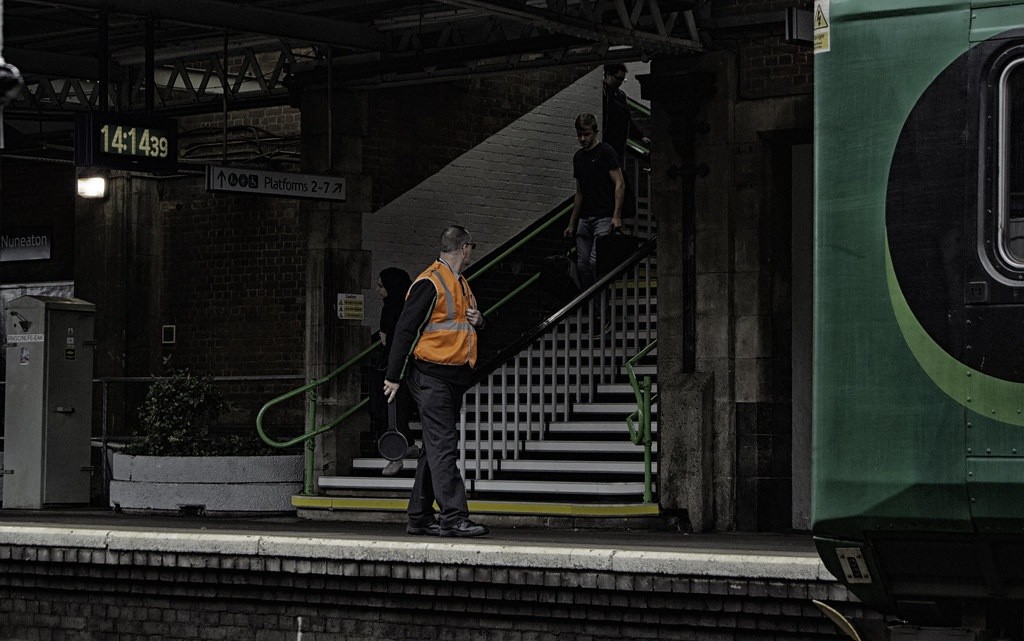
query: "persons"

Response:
[383,225,490,538]
[563,113,627,340]
[376,268,419,475]
[602,62,650,221]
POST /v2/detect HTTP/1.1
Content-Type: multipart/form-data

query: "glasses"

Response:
[615,74,625,81]
[462,243,477,250]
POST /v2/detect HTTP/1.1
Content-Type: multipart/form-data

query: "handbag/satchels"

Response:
[594,224,632,277]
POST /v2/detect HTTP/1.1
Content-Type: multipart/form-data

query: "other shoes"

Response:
[406,445,419,458]
[438,520,489,537]
[405,519,441,536]
[591,320,620,340]
[381,460,412,475]
